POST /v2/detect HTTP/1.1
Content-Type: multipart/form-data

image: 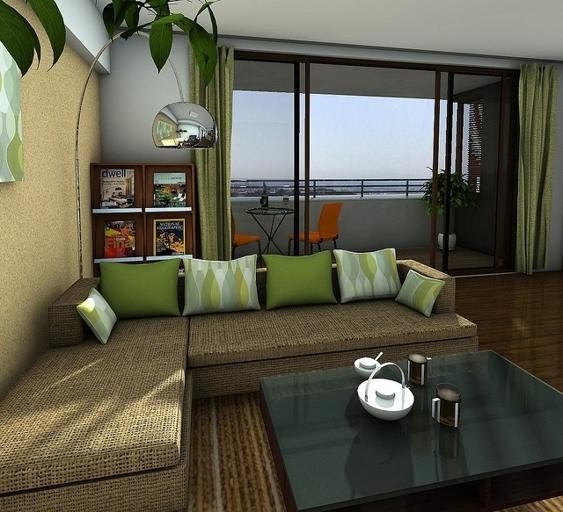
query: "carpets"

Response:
[189,390,563,511]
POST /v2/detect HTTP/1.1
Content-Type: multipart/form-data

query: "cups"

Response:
[353,357,381,378]
[431,383,461,428]
[406,352,432,386]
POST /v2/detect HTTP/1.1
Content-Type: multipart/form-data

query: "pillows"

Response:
[184,254,261,315]
[259,250,338,310]
[396,269,445,317]
[78,286,117,344]
[98,258,182,314]
[334,248,401,303]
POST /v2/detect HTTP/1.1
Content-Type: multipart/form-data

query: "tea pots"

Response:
[356,361,415,420]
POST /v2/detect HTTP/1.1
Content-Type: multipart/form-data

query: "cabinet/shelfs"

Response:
[91,163,196,278]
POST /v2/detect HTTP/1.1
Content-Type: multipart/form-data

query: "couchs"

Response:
[0,248,478,511]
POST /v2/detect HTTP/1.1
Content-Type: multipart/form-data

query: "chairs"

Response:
[231,207,263,268]
[288,202,341,255]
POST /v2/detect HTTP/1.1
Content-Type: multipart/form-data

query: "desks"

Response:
[245,207,294,256]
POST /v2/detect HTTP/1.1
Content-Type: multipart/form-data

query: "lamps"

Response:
[74,20,220,278]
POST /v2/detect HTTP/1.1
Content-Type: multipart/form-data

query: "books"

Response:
[154,218,186,256]
[104,220,136,258]
[154,171,188,208]
[101,168,135,208]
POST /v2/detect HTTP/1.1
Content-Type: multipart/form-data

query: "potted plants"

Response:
[419,167,478,249]
